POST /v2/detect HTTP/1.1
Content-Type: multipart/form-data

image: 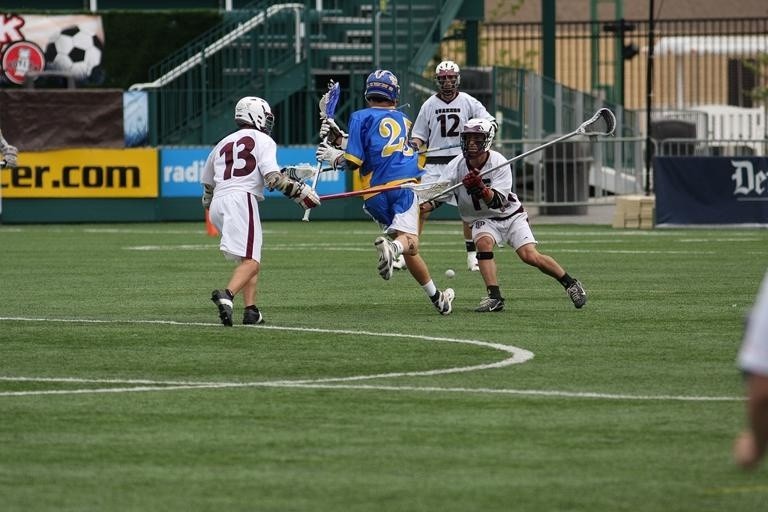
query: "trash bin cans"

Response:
[538,134,591,215]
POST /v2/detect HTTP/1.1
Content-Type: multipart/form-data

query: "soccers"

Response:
[44,25,106,81]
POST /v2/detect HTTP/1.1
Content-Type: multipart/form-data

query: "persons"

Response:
[393,60,499,272]
[732,268,768,467]
[419,117,587,312]
[0,132,18,169]
[198,96,321,327]
[319,69,456,316]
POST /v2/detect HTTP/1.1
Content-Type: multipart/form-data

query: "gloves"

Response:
[320,118,343,144]
[315,143,345,170]
[462,168,486,198]
[290,184,322,212]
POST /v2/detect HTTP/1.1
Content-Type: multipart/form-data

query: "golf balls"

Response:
[444,270,455,278]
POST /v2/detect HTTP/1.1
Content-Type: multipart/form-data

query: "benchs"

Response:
[223,1,458,82]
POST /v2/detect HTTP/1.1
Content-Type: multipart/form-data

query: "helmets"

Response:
[433,59,461,96]
[363,68,401,106]
[234,96,275,136]
[459,118,495,159]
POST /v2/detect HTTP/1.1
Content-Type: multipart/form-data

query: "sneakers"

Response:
[391,253,408,272]
[474,297,505,313]
[242,306,265,326]
[565,278,587,309]
[211,288,234,328]
[466,258,479,273]
[372,236,396,280]
[434,288,457,316]
